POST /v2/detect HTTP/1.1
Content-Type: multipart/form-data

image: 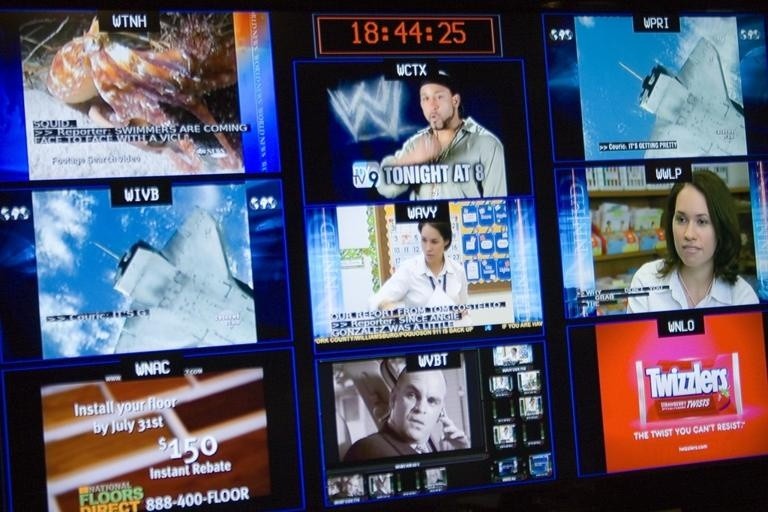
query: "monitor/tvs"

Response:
[315,338,559,512]
[552,161,768,323]
[541,7,767,162]
[292,56,535,206]
[0,6,284,186]
[0,179,294,368]
[0,345,307,512]
[564,304,768,479]
[305,196,546,358]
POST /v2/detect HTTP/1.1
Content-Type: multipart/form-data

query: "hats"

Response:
[420,71,464,116]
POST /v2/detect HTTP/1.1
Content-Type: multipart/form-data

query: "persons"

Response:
[369,218,472,325]
[376,69,507,200]
[342,366,470,460]
[626,168,760,314]
